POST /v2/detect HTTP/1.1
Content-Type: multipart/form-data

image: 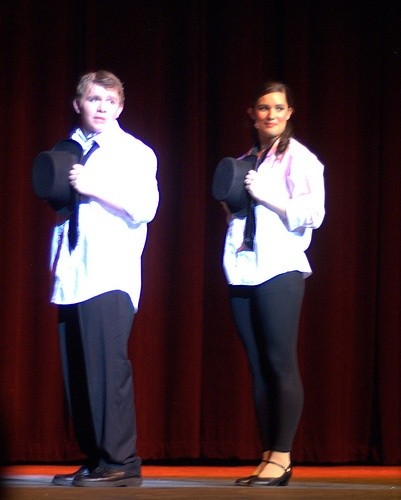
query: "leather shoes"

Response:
[75,460,144,487]
[54,465,89,485]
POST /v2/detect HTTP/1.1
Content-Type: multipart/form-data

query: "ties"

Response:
[69,143,96,250]
[243,149,269,250]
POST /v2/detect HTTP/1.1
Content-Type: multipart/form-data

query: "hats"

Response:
[212,155,257,213]
[31,138,81,223]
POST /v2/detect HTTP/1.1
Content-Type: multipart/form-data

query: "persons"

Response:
[44,70,160,491]
[216,81,328,489]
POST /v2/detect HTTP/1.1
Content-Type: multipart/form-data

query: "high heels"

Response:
[249,465,294,487]
[235,460,269,486]
